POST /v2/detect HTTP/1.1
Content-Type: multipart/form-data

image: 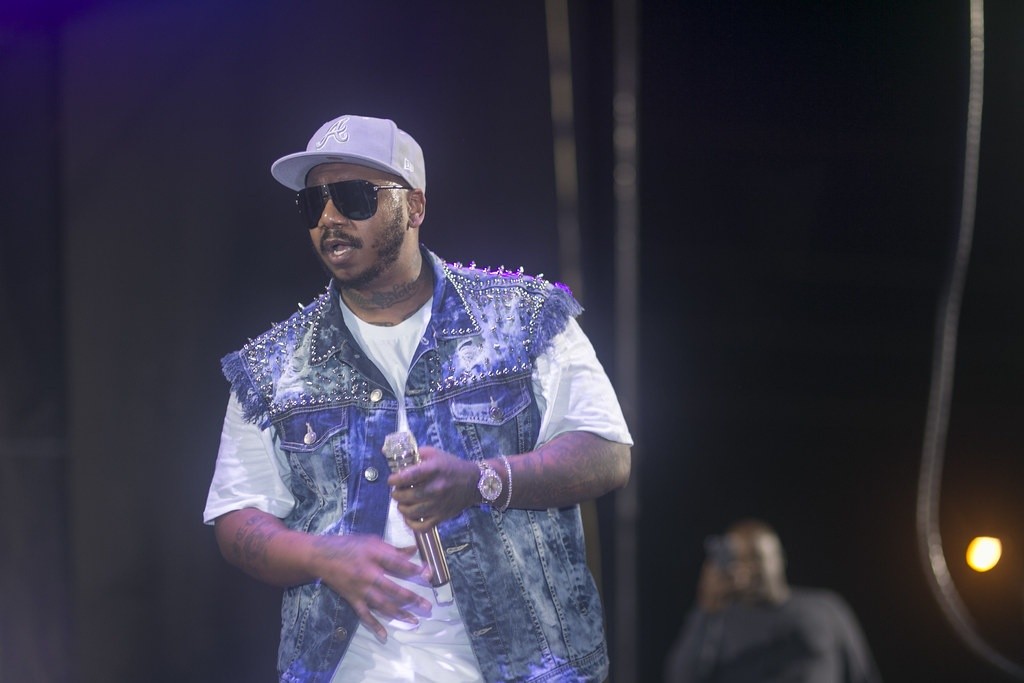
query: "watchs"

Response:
[475,460,503,504]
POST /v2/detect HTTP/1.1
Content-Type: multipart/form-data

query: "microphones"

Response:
[380,428,453,606]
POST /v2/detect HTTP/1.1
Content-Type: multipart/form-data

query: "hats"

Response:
[272,115,426,196]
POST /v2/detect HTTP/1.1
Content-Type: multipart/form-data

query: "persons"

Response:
[204,114,634,683]
[671,521,876,683]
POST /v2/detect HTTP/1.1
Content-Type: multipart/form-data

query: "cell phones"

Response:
[703,536,733,571]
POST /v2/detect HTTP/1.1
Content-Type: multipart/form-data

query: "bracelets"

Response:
[501,455,512,508]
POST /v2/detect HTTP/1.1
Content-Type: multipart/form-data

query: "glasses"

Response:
[295,179,417,229]
